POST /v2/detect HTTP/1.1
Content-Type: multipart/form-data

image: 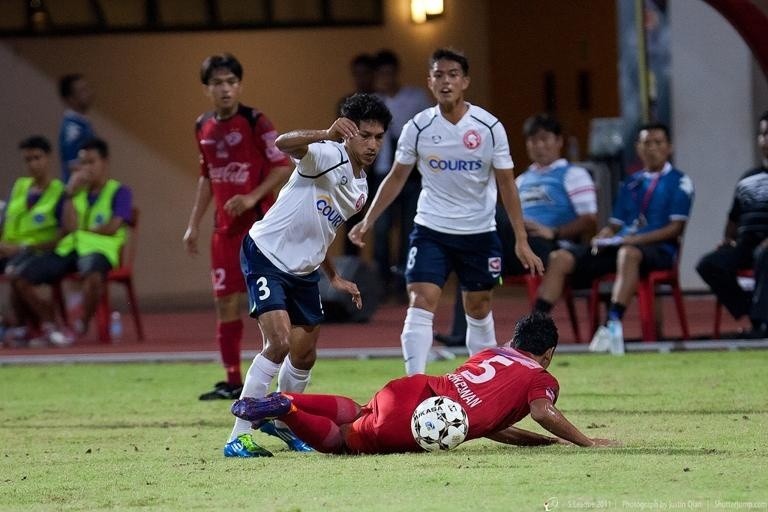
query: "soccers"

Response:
[410,395,469,451]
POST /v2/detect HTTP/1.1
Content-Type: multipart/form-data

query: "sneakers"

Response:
[4,321,86,349]
[200,381,316,456]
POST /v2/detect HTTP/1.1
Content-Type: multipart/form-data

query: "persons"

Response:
[696,111,768,338]
[2,134,77,346]
[535,121,695,354]
[335,54,378,257]
[369,48,432,303]
[223,91,392,458]
[348,48,545,379]
[57,73,97,180]
[499,115,598,315]
[232,315,618,454]
[19,138,133,348]
[182,53,295,400]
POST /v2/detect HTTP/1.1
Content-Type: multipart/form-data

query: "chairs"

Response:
[589,240,690,342]
[504,272,580,342]
[713,267,758,340]
[52,208,146,343]
